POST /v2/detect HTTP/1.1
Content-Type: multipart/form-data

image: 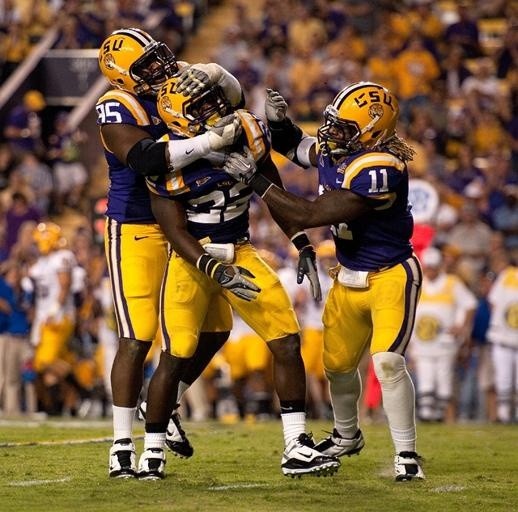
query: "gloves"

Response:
[265,88,288,122]
[204,113,258,185]
[196,254,259,301]
[171,65,219,97]
[297,246,322,300]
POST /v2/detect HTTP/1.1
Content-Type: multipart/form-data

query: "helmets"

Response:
[31,221,61,254]
[98,28,178,97]
[317,81,401,156]
[157,77,232,137]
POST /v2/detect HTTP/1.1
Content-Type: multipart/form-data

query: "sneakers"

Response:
[108,402,193,480]
[393,452,425,482]
[281,428,365,478]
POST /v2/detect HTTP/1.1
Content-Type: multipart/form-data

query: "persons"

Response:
[96,27,246,481]
[0,0,518,424]
[137,67,341,483]
[222,81,426,483]
